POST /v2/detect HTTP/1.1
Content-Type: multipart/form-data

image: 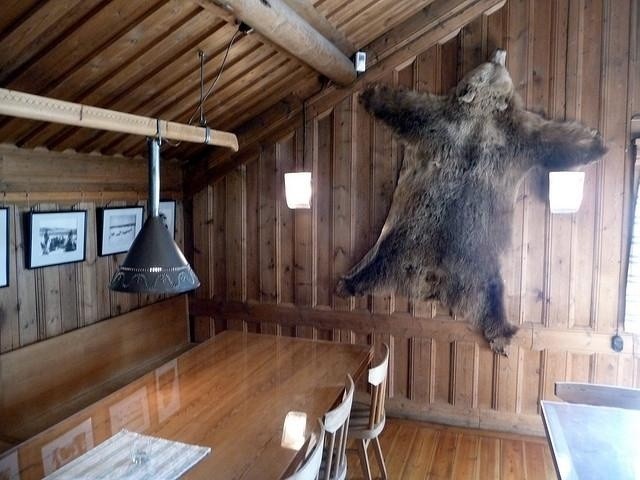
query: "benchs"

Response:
[0,295,201,458]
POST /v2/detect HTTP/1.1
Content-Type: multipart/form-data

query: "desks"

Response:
[539,401,638,480]
[0,328,376,480]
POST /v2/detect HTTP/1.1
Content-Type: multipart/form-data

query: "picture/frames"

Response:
[29,209,88,270]
[0,449,22,480]
[106,384,152,437]
[0,206,10,288]
[153,358,182,426]
[159,199,175,240]
[99,205,145,257]
[38,414,97,478]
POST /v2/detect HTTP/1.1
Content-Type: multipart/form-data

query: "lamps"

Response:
[109,133,202,296]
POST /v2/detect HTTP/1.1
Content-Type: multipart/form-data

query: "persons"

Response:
[42,228,50,255]
[65,230,76,251]
[50,235,65,252]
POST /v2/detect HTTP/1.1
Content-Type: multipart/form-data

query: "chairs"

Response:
[554,382,640,411]
[335,339,391,480]
[282,416,327,480]
[324,372,357,480]
